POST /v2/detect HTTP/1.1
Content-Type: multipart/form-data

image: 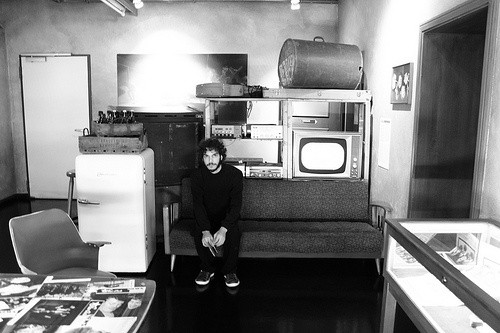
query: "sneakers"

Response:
[195,270,214,285]
[224,273,240,288]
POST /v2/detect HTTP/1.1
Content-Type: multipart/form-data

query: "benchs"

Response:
[162,176,387,275]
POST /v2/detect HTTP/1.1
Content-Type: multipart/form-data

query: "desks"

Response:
[0,273,156,333]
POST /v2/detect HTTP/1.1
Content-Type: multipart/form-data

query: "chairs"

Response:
[9,208,118,277]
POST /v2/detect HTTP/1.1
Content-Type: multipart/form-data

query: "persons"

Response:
[190,137,243,287]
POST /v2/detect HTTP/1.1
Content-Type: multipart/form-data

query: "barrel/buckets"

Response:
[278,35,363,90]
[108,105,206,185]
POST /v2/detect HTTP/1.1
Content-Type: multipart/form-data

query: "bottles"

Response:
[97,110,136,124]
